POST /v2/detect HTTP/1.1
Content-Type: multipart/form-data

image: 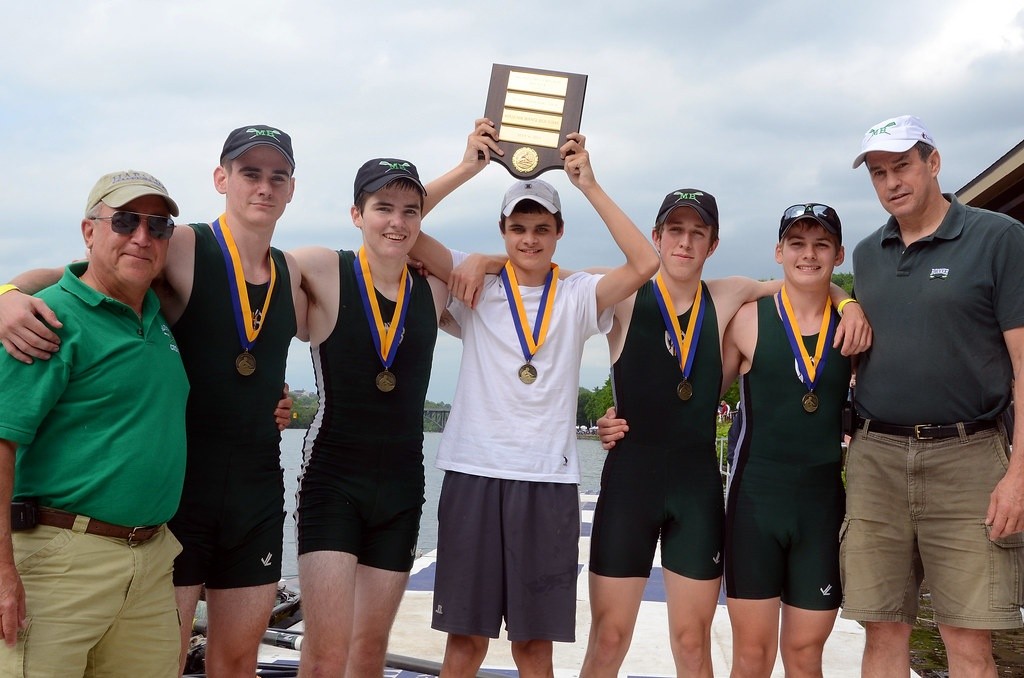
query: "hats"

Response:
[779,203,843,246]
[219,126,295,175]
[353,158,428,204]
[85,171,179,217]
[501,178,561,218]
[851,118,935,170]
[655,189,718,227]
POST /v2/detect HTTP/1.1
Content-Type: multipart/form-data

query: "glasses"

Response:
[98,210,176,241]
[782,204,841,237]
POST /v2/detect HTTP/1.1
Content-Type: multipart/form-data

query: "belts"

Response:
[34,506,161,547]
[855,416,1001,441]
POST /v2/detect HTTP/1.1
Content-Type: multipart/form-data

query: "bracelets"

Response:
[838,299,857,317]
[0,284,23,296]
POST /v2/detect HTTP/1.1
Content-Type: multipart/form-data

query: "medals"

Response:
[236,353,256,375]
[677,381,692,400]
[519,364,537,384]
[802,393,818,412]
[376,371,396,392]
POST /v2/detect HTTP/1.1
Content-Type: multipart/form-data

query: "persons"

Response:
[284,158,462,678]
[0,171,293,678]
[718,401,730,423]
[446,189,872,678]
[0,125,428,678]
[839,116,1024,678]
[407,118,662,678]
[596,203,859,678]
